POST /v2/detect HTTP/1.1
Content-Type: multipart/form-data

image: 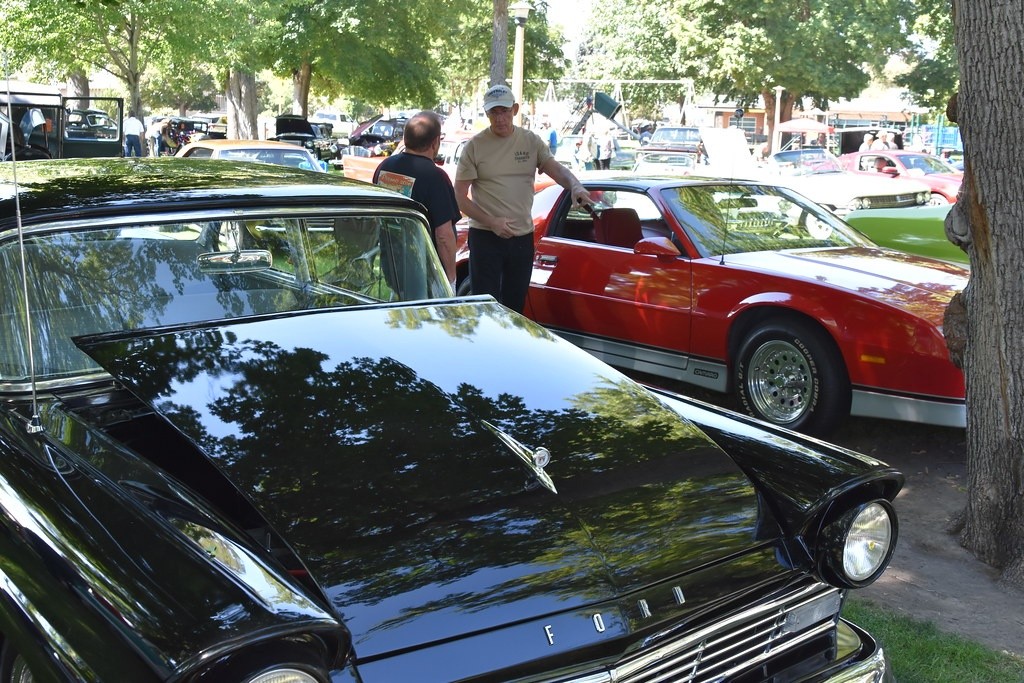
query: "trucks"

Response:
[0,80,124,160]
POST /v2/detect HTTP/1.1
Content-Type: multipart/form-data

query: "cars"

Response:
[556,124,965,213]
[67,109,406,173]
[454,172,971,440]
[0,157,904,682]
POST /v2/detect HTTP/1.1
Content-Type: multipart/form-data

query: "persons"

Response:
[374,111,462,302]
[148,117,157,130]
[123,111,145,157]
[145,118,171,157]
[578,127,619,170]
[640,124,653,145]
[541,120,557,155]
[859,131,898,172]
[178,123,188,132]
[819,133,827,147]
[453,84,594,312]
[921,149,953,164]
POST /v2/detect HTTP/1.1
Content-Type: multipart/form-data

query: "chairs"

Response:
[0,290,298,371]
[596,208,644,250]
[872,158,887,172]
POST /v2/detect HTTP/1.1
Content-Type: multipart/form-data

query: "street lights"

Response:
[507,1,536,126]
[772,86,787,154]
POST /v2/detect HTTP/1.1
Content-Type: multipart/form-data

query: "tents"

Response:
[776,118,829,161]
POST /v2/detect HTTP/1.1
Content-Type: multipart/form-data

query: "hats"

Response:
[483,85,515,111]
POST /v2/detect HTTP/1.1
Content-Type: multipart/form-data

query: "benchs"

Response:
[0,242,242,313]
